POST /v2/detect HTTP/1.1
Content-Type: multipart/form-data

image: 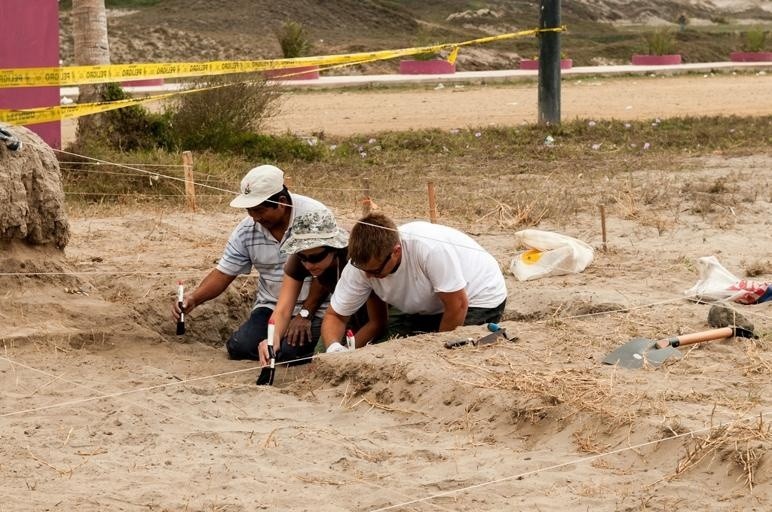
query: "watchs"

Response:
[298,309,316,322]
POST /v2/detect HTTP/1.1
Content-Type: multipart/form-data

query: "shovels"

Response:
[488,323,519,342]
[601,324,760,371]
[444,327,507,349]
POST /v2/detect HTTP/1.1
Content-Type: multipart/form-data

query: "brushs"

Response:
[255,320,275,385]
[176,282,185,335]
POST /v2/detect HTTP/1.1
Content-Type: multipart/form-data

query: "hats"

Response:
[228,164,286,209]
[277,206,350,256]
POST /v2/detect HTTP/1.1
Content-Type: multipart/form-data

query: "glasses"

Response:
[350,252,390,278]
[294,247,333,264]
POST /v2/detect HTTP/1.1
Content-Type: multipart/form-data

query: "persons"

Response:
[321,213,508,353]
[171,164,339,361]
[257,209,386,367]
